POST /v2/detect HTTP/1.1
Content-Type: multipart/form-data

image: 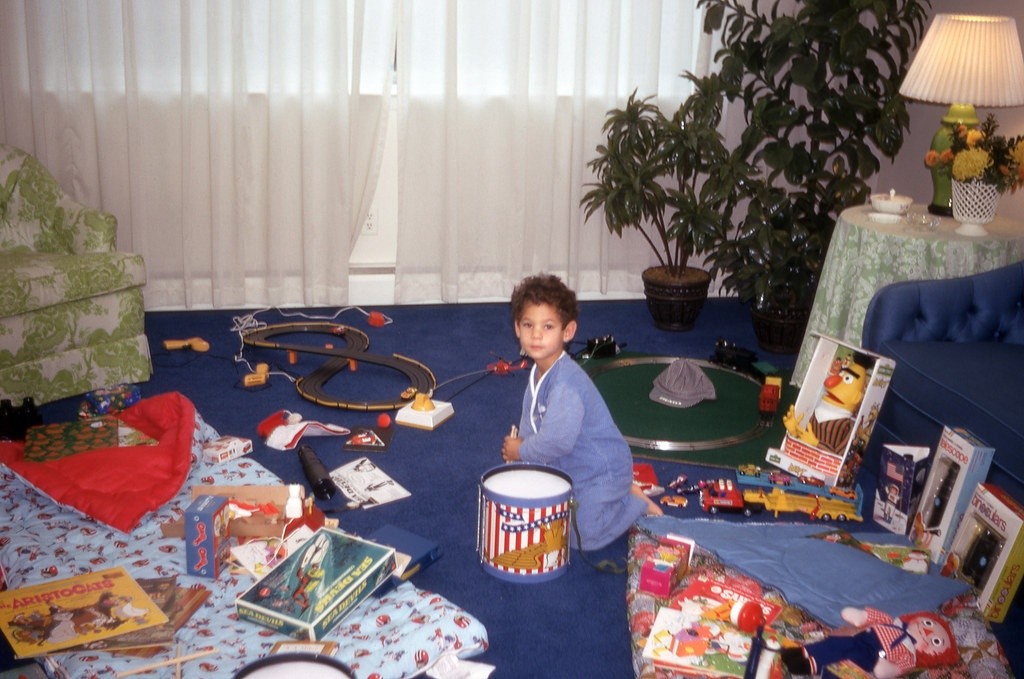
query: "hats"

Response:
[650,358,717,409]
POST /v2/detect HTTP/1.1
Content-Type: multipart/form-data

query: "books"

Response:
[0,565,213,660]
[22,414,159,462]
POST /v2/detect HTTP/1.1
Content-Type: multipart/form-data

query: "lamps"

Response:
[899,13,968,228]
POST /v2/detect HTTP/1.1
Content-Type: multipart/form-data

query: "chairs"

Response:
[0,141,153,411]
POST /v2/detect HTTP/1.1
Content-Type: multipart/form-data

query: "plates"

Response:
[869,212,902,225]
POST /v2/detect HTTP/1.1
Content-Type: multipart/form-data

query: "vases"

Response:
[952,177,1000,238]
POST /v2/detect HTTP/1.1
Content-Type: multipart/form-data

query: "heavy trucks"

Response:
[699,463,865,524]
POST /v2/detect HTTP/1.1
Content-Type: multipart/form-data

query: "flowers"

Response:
[924,110,1024,198]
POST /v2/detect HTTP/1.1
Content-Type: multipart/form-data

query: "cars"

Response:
[659,495,689,509]
[797,475,825,488]
[767,472,792,487]
[830,486,857,500]
[736,463,762,478]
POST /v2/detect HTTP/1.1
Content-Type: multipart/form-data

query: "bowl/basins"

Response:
[870,193,914,216]
[906,213,941,231]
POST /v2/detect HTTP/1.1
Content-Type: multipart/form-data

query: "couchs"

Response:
[862,260,1024,679]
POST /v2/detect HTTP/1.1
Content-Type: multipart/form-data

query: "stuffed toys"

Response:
[780,606,961,679]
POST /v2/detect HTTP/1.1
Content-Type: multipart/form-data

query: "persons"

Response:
[501,271,664,552]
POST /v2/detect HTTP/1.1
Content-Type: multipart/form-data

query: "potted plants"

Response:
[577,0,934,359]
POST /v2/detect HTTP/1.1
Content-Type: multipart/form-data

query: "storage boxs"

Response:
[185,480,445,645]
[940,482,1024,624]
[908,423,996,566]
[475,464,576,584]
[765,330,897,489]
[81,383,141,415]
[873,444,932,535]
[639,532,694,599]
[201,434,254,465]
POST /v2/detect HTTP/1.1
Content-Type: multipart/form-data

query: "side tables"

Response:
[787,202,1024,392]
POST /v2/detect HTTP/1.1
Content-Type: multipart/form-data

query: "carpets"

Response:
[625,515,1016,679]
[0,389,489,679]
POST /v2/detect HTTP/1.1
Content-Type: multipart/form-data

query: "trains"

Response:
[759,376,783,417]
